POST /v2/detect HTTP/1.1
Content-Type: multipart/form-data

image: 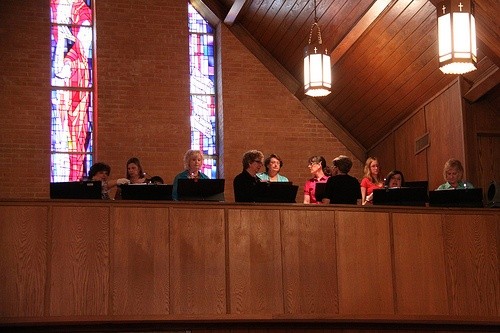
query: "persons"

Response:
[172,149,209,200]
[257,154,289,182]
[360,157,385,204]
[436,159,474,189]
[233,150,264,202]
[382,169,403,187]
[114,158,147,200]
[303,155,331,203]
[322,155,362,205]
[83,163,130,200]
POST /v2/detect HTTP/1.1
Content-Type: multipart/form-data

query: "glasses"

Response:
[254,159,263,164]
[309,162,317,166]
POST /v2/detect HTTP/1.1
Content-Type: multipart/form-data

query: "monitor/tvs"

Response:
[252,182,292,202]
[176,179,225,202]
[122,183,173,201]
[428,188,485,208]
[373,186,427,206]
[49,182,103,199]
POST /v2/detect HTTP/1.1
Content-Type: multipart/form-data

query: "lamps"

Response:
[304,0,332,97]
[437,0,478,75]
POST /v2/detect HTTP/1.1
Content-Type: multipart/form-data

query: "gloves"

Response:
[116,178,130,185]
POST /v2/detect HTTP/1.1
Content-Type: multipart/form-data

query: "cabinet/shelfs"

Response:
[0,199,500,323]
